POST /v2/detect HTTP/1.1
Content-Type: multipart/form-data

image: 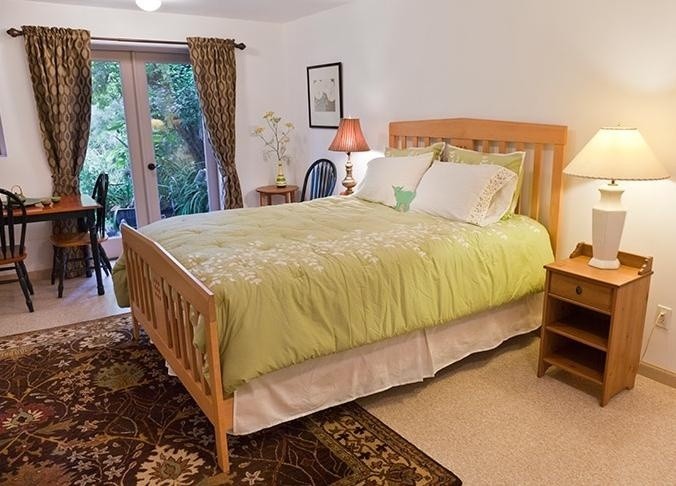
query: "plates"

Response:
[2,197,45,209]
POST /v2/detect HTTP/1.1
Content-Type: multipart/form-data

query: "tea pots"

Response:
[6,184,26,205]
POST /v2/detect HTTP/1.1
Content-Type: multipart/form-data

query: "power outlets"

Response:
[655,305,671,331]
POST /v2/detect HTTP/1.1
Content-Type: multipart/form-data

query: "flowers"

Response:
[253,112,294,163]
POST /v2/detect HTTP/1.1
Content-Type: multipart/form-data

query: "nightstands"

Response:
[536,244,654,410]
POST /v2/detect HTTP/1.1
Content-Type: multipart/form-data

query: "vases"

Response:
[275,160,288,188]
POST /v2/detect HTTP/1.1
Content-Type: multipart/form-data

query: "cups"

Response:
[51,196,61,203]
[41,200,50,207]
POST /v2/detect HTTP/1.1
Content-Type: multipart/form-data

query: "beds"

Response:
[111,118,566,470]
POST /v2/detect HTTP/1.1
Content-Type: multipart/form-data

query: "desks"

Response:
[0,193,106,295]
[256,185,299,206]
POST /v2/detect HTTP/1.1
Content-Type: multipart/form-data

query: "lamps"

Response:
[561,123,671,269]
[134,0,162,12]
[330,117,372,196]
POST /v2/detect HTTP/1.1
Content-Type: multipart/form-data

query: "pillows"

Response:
[410,159,517,226]
[444,144,526,217]
[356,152,435,210]
[385,143,444,162]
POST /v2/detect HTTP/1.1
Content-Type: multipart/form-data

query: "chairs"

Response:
[0,188,36,312]
[49,170,113,297]
[300,159,336,201]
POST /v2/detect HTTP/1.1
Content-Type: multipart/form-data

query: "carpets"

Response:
[0,313,461,486]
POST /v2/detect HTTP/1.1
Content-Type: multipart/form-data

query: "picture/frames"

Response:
[306,61,344,129]
